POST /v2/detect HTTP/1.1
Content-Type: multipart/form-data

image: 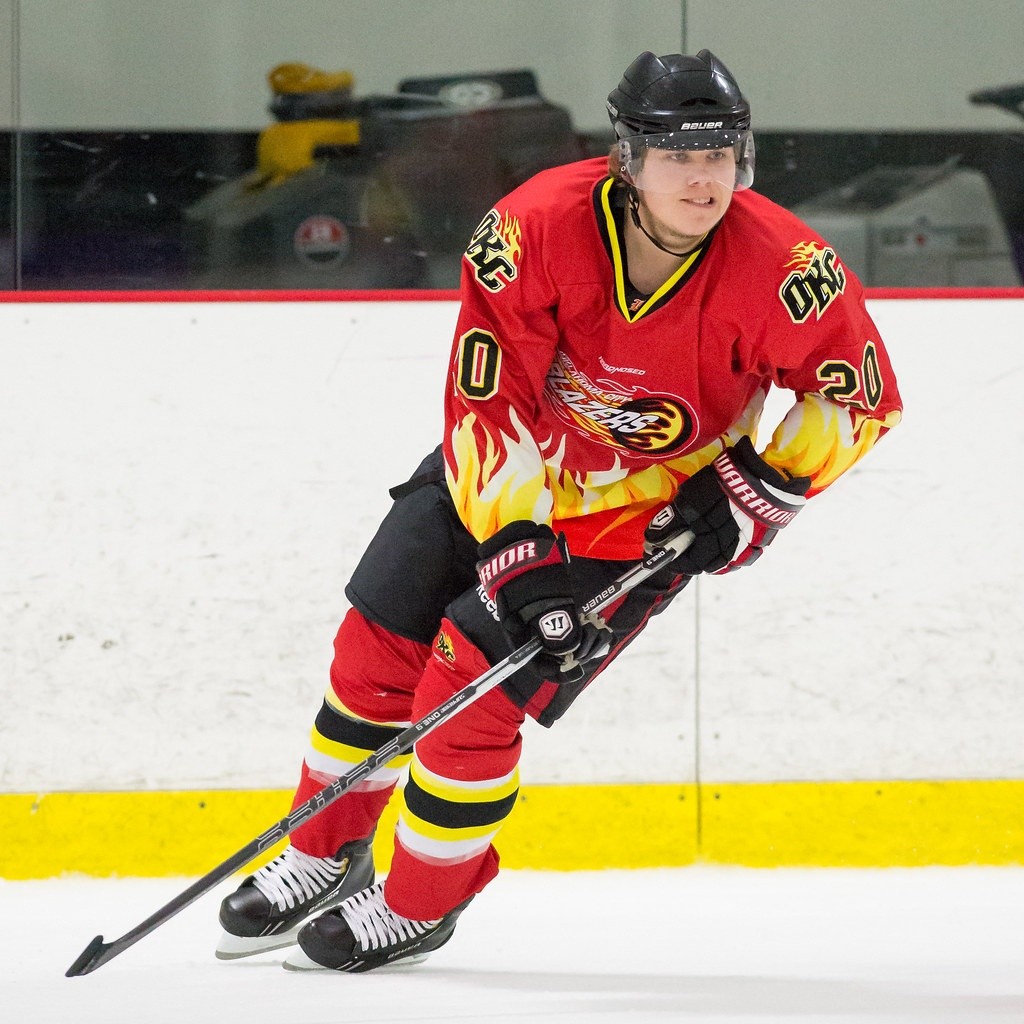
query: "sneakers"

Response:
[214,832,375,960]
[282,880,476,973]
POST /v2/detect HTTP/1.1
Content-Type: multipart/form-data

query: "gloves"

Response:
[475,520,619,685]
[644,434,811,576]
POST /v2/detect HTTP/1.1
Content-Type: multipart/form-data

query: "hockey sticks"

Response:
[62,527,696,981]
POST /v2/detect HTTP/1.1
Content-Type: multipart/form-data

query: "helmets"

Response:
[605,48,756,194]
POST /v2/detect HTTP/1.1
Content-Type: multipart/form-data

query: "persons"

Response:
[209,51,902,981]
